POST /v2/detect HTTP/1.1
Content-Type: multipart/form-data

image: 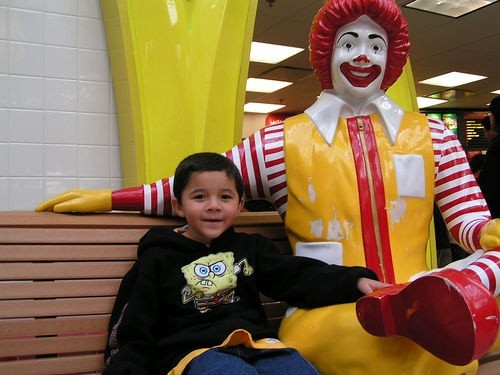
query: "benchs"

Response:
[0,211,288,375]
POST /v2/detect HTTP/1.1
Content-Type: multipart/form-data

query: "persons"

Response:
[434,95,500,269]
[102,152,395,375]
[34,0,500,375]
